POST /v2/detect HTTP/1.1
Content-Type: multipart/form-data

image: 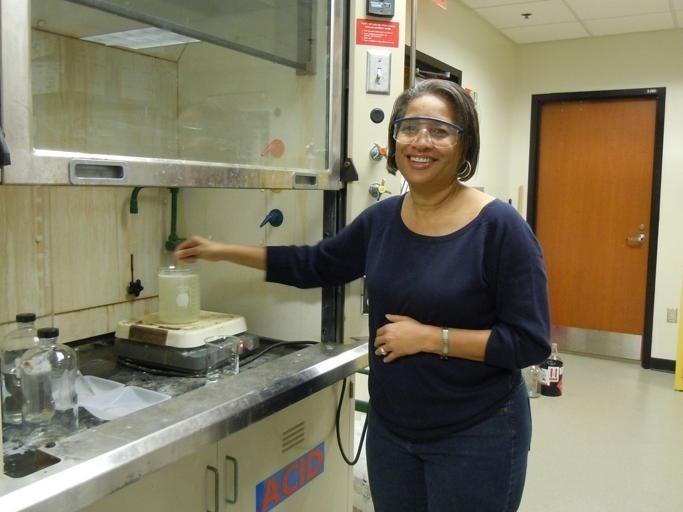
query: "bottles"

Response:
[0,312,87,448]
[523,341,569,398]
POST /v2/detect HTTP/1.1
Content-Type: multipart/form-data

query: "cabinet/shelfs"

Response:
[82,375,356,510]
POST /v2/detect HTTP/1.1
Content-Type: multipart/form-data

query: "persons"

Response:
[173,78,551,512]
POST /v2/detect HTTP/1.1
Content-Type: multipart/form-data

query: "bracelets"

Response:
[441,326,449,359]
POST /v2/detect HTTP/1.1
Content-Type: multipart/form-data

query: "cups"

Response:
[156,263,203,326]
[202,334,242,386]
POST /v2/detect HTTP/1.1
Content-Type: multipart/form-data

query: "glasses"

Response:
[393,117,464,150]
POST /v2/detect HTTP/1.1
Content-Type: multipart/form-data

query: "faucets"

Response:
[128,186,186,252]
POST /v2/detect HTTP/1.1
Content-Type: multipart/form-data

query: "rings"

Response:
[381,347,387,356]
